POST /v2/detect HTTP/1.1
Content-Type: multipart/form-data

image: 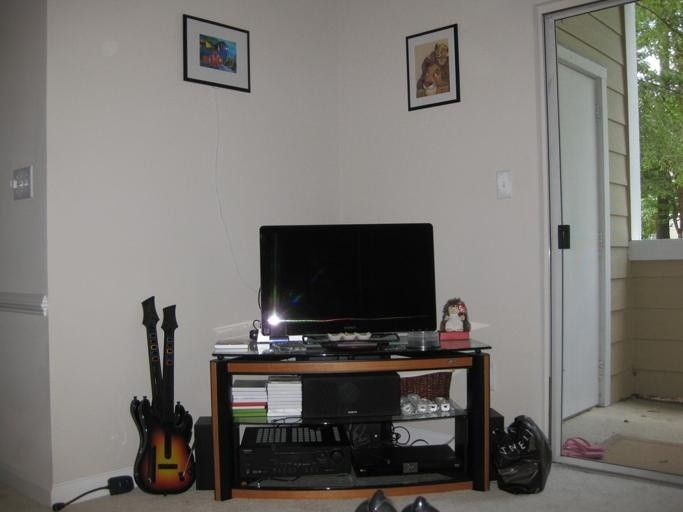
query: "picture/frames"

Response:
[406,24,460,111]
[183,13,250,92]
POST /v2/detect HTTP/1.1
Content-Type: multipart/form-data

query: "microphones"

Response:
[53,476,134,512]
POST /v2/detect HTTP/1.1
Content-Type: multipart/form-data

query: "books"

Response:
[213,339,250,349]
[231,375,303,418]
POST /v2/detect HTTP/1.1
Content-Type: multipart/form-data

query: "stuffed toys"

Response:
[439,296,471,332]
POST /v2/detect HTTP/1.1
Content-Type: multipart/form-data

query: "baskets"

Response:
[399,371,451,400]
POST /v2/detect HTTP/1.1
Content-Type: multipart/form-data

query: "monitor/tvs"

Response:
[258,224,438,349]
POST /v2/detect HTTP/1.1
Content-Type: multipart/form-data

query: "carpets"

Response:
[579,433,683,477]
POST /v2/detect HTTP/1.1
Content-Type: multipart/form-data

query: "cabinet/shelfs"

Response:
[210,339,492,501]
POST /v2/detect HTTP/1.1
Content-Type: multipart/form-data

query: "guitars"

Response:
[130,296,195,496]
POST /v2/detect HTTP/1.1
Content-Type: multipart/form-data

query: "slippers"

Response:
[561,437,605,459]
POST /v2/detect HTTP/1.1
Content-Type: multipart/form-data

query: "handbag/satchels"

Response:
[494,415,552,495]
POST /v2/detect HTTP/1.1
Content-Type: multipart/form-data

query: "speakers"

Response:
[193,415,241,491]
[455,407,503,481]
[302,372,401,416]
